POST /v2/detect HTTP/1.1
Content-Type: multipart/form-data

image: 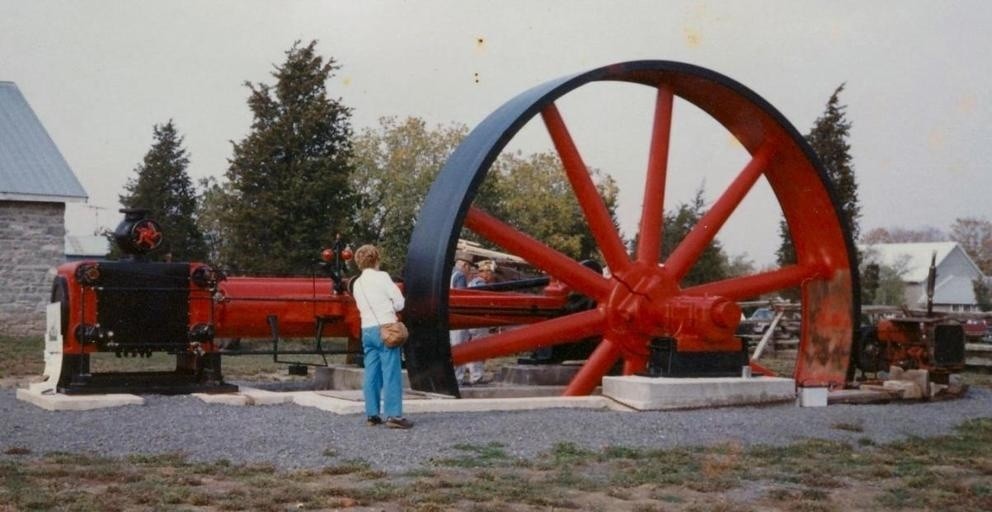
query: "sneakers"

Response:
[385,416,417,428]
[457,374,496,387]
[366,415,382,426]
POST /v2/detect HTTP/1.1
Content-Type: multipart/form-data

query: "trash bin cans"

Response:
[934,326,965,375]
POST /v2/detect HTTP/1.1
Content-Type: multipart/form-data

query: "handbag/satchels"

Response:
[379,320,410,350]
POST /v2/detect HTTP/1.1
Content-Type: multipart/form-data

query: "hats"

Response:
[452,252,481,267]
[478,259,496,272]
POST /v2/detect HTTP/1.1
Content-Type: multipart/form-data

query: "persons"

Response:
[467,263,495,383]
[448,251,473,387]
[348,244,417,427]
